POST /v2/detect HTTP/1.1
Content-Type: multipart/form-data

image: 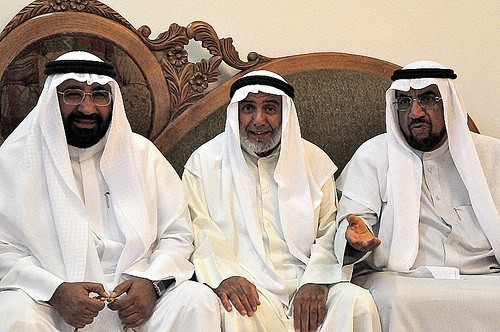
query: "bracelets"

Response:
[151,280,166,299]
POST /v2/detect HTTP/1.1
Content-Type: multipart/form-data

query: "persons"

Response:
[336,60,500,331]
[181,68,383,332]
[0,50,221,332]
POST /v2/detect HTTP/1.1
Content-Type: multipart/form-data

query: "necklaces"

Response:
[73,294,140,332]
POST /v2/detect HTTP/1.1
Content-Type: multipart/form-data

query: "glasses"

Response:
[58,89,112,106]
[393,95,442,112]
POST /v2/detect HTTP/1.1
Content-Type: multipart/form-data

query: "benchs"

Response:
[0,0,481,204]
